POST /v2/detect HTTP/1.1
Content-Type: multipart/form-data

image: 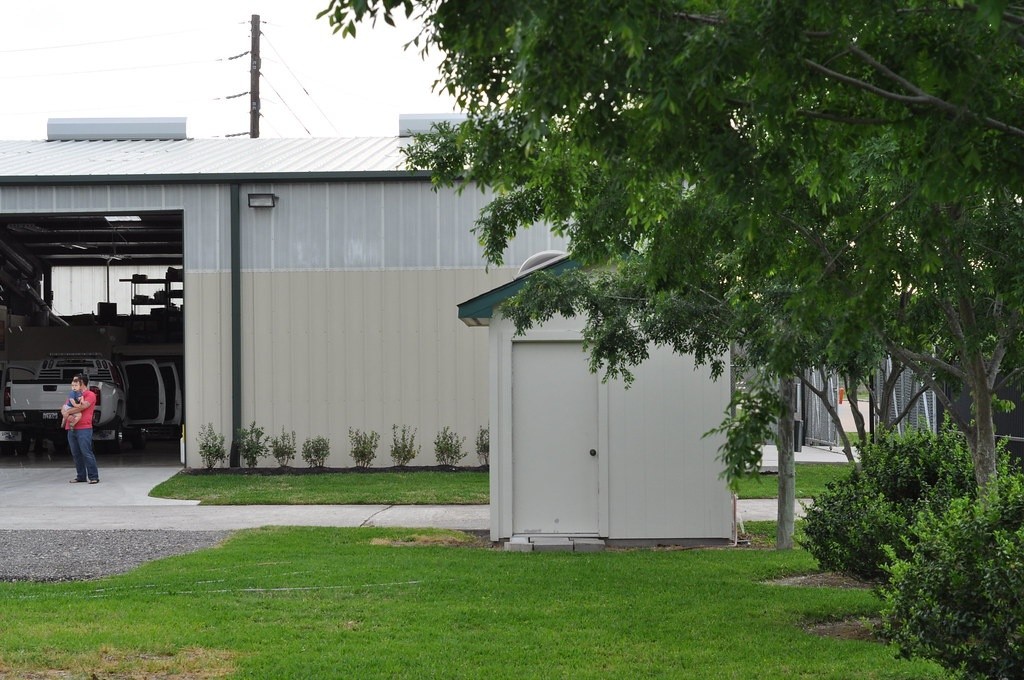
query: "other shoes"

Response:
[89,478,99,484]
[70,479,87,483]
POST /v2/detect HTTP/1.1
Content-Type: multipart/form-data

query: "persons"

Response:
[61,374,99,484]
[61,379,85,432]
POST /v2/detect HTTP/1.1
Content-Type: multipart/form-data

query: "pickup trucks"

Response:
[0,352,182,454]
